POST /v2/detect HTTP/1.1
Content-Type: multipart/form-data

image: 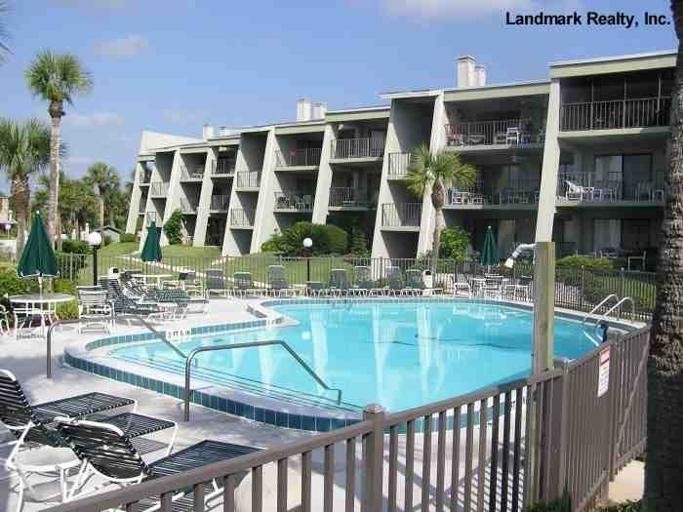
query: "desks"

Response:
[8,294,76,340]
[506,127,519,145]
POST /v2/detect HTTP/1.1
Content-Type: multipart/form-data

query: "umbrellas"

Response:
[141,218,163,275]
[480,226,499,273]
[18,210,62,327]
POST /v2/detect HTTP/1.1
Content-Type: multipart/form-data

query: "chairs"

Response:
[563,179,620,202]
[0,296,55,341]
[632,178,653,204]
[75,264,210,336]
[203,264,298,299]
[293,194,313,209]
[453,271,531,304]
[305,262,445,297]
[0,366,272,512]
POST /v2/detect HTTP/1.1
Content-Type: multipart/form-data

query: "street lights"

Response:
[303,238,312,282]
[89,232,103,315]
